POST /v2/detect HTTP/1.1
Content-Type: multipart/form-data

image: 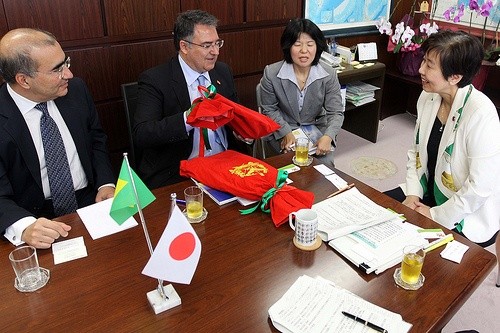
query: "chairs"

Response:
[121,80,268,159]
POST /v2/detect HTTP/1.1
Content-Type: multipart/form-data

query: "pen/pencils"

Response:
[327,183,355,198]
[330,43,338,49]
[423,233,453,250]
[424,236,454,252]
[342,311,388,333]
[288,144,295,148]
[169,197,186,204]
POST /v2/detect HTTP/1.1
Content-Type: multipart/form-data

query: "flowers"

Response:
[374,0,500,60]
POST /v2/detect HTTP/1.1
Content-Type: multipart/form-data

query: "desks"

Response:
[335,61,386,143]
[0,154,497,333]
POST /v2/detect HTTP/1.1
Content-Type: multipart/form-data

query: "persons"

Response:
[131,11,254,192]
[260,18,344,169]
[382,28,500,247]
[0,27,116,249]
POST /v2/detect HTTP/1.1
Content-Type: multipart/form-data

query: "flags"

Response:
[140,205,201,284]
[110,157,156,225]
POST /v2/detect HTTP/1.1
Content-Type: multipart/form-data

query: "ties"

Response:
[33,102,78,216]
[198,76,213,151]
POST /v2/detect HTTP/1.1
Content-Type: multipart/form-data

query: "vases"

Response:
[472,60,496,92]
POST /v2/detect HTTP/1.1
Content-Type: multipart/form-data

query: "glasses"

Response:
[182,38,224,48]
[28,57,71,79]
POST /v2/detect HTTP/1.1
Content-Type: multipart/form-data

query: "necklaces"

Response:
[297,77,305,83]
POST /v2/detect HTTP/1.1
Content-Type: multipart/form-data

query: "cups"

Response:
[184,186,205,220]
[295,137,310,163]
[288,209,319,245]
[8,245,42,288]
[400,245,426,284]
